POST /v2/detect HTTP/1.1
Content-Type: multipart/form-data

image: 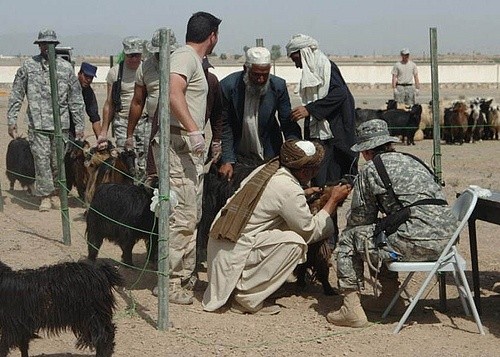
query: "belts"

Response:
[397,83,413,87]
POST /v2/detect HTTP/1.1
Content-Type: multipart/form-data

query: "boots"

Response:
[369,279,405,313]
[326,291,368,328]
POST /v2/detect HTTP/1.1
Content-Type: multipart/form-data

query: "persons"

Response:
[326,119,459,329]
[391,48,420,109]
[5,12,356,315]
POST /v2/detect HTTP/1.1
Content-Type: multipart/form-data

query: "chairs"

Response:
[380,187,486,334]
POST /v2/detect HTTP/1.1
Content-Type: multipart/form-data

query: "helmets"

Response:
[401,48,409,54]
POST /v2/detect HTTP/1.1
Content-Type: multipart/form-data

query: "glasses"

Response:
[126,53,142,57]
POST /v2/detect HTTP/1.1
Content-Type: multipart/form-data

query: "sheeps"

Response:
[85,164,234,268]
[0,259,126,357]
[355,95,500,146]
[288,178,354,295]
[6,137,131,215]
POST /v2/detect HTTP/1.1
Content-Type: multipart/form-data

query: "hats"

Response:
[246,47,271,64]
[146,28,176,53]
[203,55,215,69]
[351,119,399,152]
[33,29,59,45]
[295,140,316,156]
[286,34,317,57]
[122,36,143,54]
[81,62,97,78]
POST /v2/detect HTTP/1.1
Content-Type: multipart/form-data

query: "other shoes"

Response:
[230,302,281,314]
[39,197,51,212]
[153,280,193,304]
[181,276,208,292]
